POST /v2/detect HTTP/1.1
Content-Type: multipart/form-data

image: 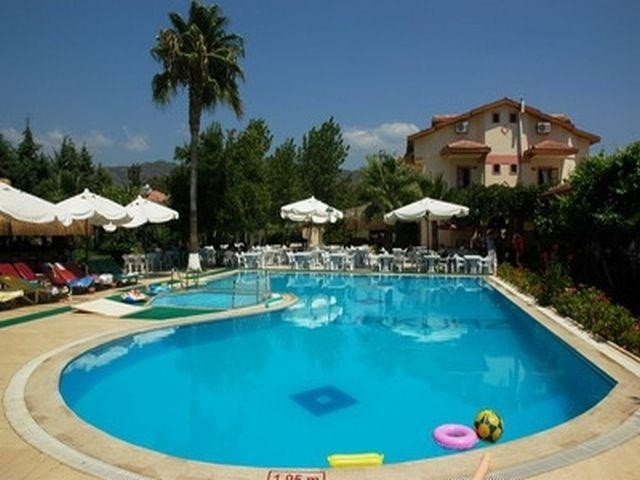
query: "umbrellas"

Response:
[280,195,343,248]
[281,294,343,329]
[384,198,470,251]
[0,181,179,263]
[382,315,468,343]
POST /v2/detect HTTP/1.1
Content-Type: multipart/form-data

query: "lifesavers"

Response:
[433,424,477,449]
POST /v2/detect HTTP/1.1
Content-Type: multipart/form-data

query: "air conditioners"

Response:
[455,121,469,133]
[537,121,551,133]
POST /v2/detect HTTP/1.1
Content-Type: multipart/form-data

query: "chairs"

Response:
[185,253,204,289]
[203,241,500,275]
[0,258,101,308]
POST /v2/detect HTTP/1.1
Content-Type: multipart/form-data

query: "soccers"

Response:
[474,410,503,442]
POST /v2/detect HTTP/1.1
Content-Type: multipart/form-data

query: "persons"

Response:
[470,229,561,271]
